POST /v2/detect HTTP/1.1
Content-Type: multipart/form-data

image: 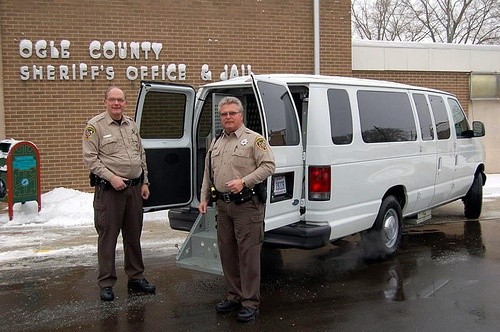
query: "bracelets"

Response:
[142,182,150,187]
[241,179,248,188]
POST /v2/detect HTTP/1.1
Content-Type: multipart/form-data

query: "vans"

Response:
[133,73,488,277]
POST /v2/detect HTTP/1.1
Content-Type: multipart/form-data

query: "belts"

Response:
[124,179,141,186]
[215,187,258,203]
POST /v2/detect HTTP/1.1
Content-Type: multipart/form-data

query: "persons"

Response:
[198,96,277,322]
[82,87,158,302]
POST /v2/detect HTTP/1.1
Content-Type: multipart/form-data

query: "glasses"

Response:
[220,111,244,117]
[106,98,126,103]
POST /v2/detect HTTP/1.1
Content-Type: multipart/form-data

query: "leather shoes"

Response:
[100,287,114,300]
[127,278,156,293]
[216,297,240,312]
[237,307,259,321]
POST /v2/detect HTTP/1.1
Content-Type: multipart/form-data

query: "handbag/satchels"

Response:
[258,183,267,200]
[89,172,98,187]
